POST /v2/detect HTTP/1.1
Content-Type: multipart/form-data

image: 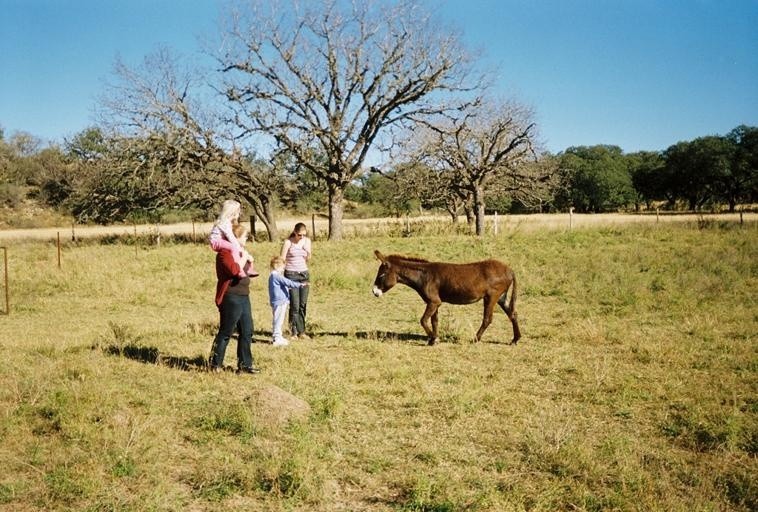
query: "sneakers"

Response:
[248,270,259,277]
[238,272,246,278]
[273,333,310,345]
[237,368,260,374]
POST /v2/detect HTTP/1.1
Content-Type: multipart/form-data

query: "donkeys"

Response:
[371,250,522,346]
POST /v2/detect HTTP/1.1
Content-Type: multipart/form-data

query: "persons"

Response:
[209,199,259,278]
[208,226,260,374]
[268,256,311,345]
[279,223,312,338]
